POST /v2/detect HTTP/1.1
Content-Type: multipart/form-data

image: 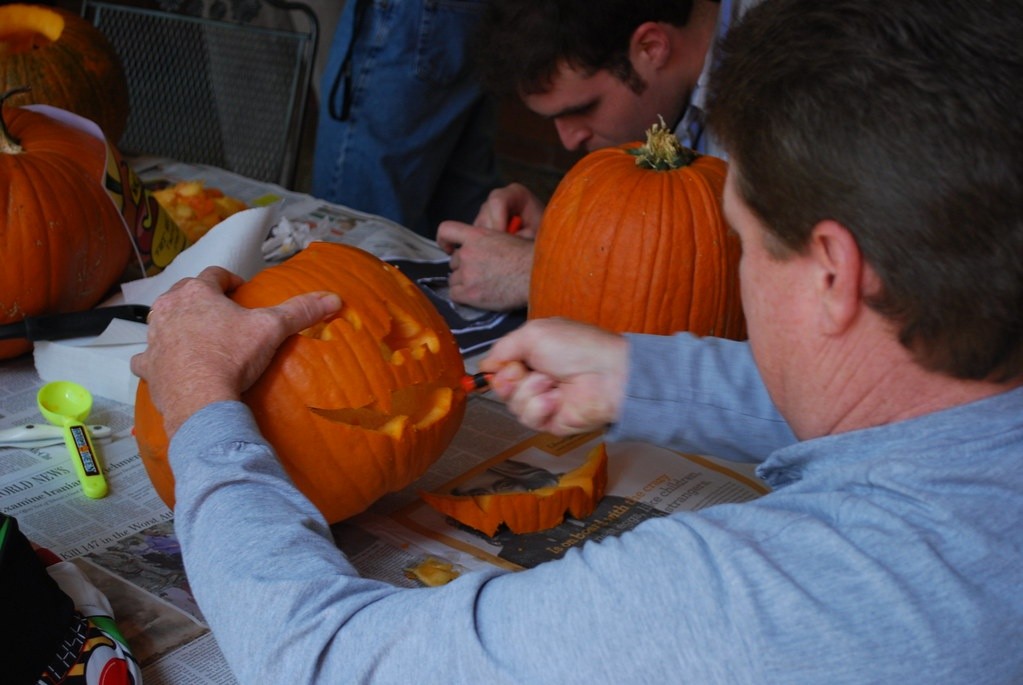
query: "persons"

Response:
[130,0,1023,685]
[0,511,142,685]
[312,0,762,312]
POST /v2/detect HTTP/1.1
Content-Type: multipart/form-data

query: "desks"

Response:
[0,148,772,685]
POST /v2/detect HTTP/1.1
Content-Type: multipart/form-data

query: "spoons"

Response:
[36,380,108,499]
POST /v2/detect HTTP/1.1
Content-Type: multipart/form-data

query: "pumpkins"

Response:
[527,114,749,341]
[0,5,127,357]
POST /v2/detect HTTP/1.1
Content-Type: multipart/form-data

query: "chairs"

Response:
[77,0,318,194]
